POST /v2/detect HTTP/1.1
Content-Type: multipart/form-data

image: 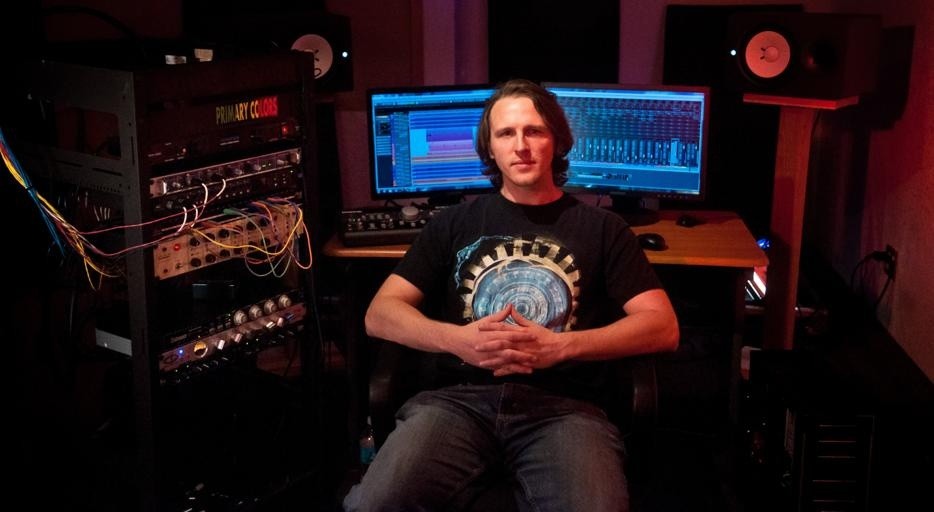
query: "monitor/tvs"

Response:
[540,82,712,227]
[365,82,498,206]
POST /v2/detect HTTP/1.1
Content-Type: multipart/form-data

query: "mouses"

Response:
[638,233,665,251]
[677,215,698,227]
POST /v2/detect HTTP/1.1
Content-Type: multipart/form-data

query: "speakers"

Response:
[728,10,889,101]
[208,13,353,94]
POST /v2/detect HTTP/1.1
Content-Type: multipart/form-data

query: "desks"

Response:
[316,204,768,468]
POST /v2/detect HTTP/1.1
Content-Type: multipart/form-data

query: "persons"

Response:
[340,78,680,510]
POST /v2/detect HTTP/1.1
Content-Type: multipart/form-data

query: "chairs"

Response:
[365,310,668,508]
